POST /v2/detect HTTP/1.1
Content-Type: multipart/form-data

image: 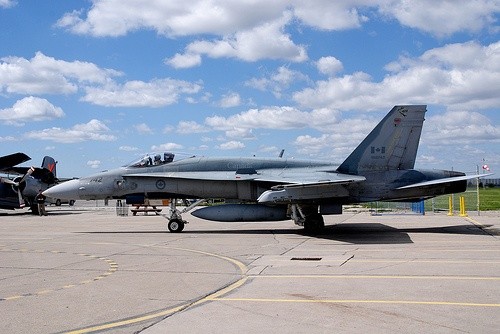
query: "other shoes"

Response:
[44,214,47,216]
[39,213,42,216]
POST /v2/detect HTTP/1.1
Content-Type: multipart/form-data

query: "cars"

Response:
[44,197,76,206]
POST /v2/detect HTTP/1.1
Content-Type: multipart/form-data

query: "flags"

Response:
[483,159,490,171]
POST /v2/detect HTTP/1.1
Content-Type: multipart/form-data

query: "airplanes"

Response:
[0,153,61,215]
[40,104,494,232]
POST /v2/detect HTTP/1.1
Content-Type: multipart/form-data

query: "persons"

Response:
[153,154,162,165]
